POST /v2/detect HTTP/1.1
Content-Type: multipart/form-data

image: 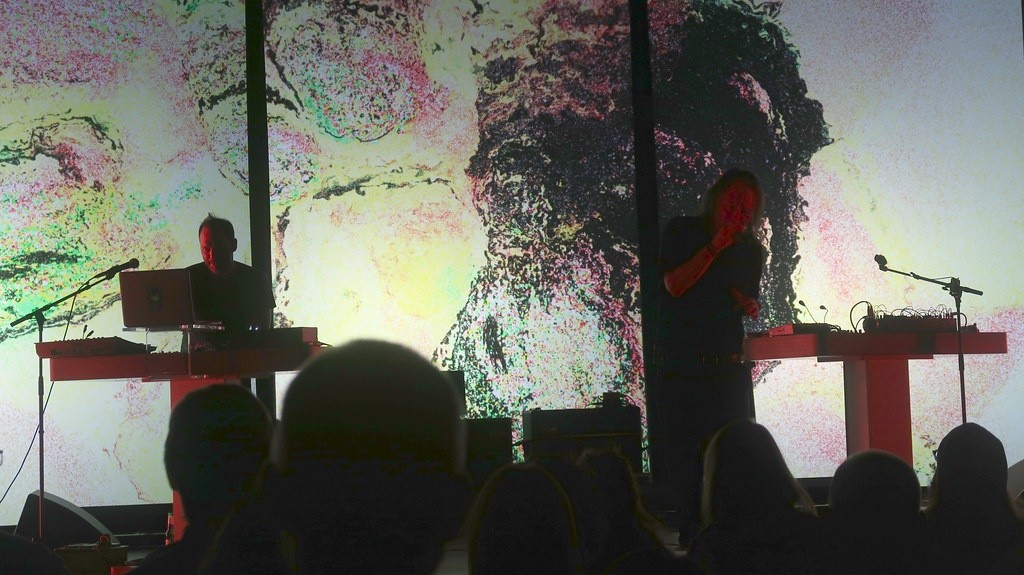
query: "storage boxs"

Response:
[464,418,512,500]
[523,405,643,482]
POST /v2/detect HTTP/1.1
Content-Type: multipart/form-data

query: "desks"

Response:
[738,331,1008,470]
[50,346,330,541]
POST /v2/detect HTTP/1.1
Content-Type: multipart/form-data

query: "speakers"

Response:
[13,490,119,548]
[523,405,643,481]
[1006,459,1024,522]
[463,417,511,474]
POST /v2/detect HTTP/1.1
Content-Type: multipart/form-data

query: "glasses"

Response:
[932,448,946,462]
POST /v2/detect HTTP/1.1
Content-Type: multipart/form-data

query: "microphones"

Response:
[799,300,817,324]
[735,233,746,244]
[820,305,828,323]
[95,259,140,278]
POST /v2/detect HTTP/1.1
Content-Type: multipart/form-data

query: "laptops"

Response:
[119,269,222,328]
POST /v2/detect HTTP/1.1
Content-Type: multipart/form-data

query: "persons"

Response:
[170,217,276,545]
[658,169,766,547]
[123,339,1023,574]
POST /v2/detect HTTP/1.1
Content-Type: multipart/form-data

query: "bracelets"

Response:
[707,243,718,256]
